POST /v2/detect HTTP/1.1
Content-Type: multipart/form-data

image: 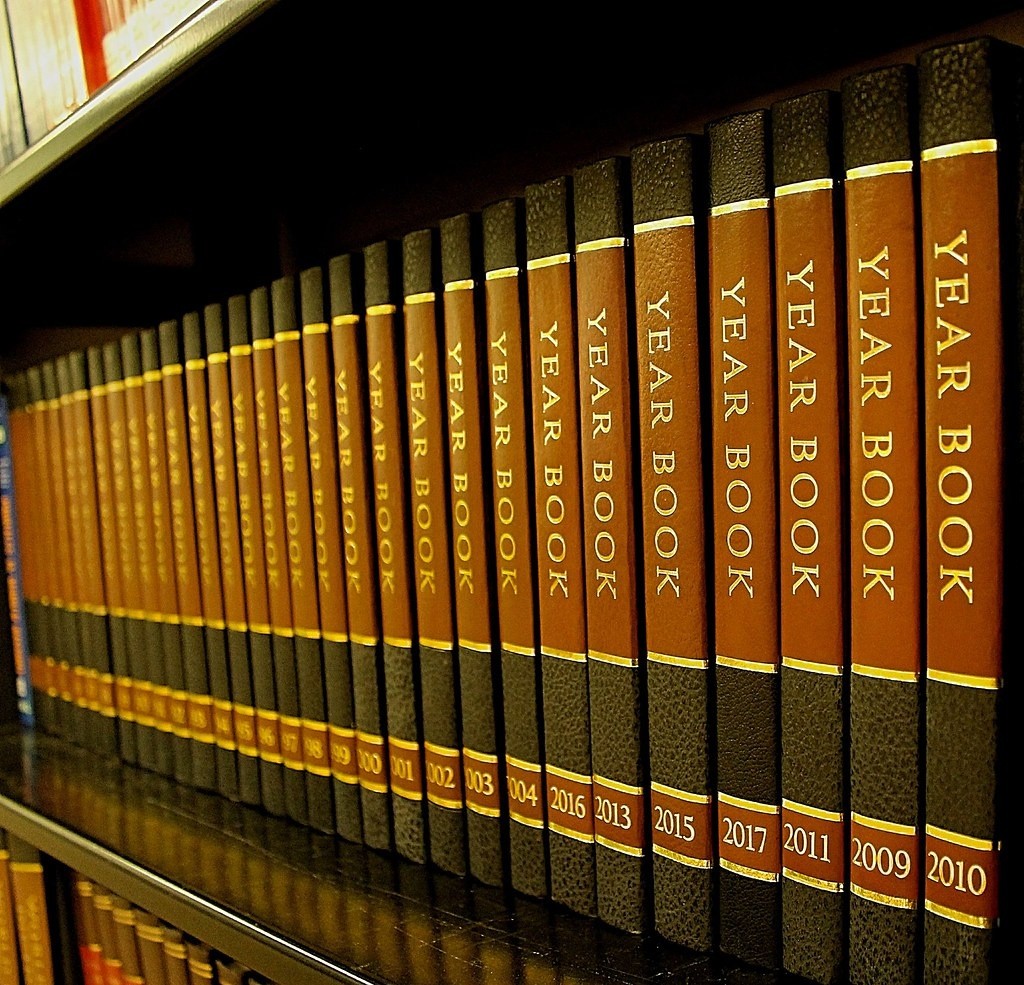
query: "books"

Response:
[0,0,210,170]
[1,34,1024,984]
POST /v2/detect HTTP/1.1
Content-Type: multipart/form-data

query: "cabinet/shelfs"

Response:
[0,0,891,985]
